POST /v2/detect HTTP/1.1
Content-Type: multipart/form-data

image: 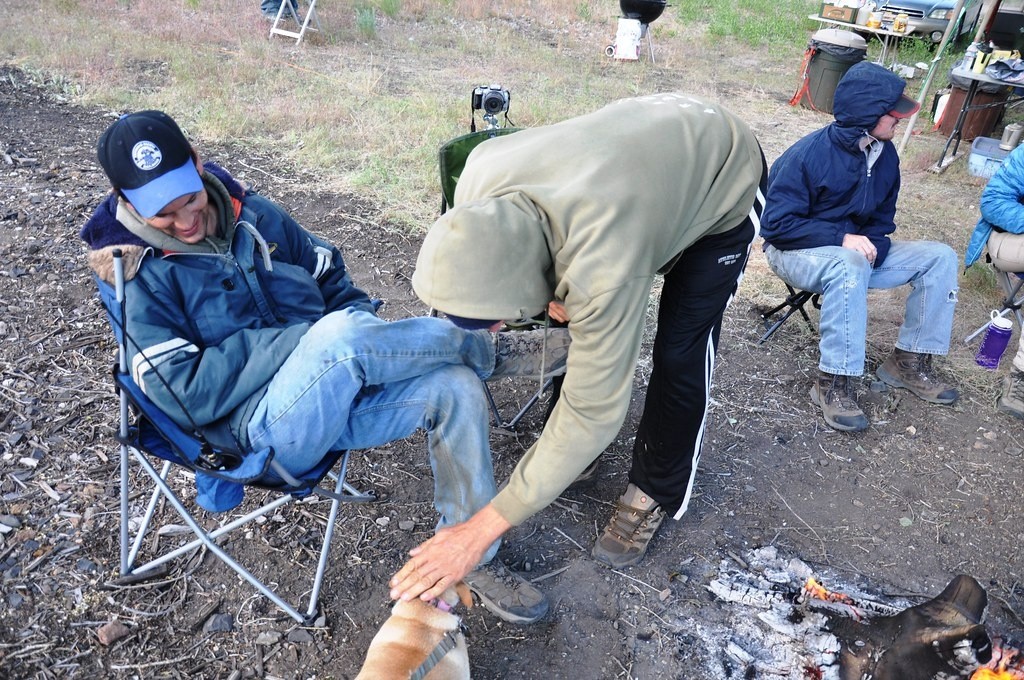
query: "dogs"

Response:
[356,580,473,680]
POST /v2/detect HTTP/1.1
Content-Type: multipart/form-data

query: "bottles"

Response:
[971,46,993,73]
[856,0,872,25]
[976,310,1013,370]
[893,14,909,33]
[960,41,977,71]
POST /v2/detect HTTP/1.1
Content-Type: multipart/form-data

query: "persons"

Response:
[80,109,549,624]
[387,93,768,603]
[260,0,311,26]
[965,142,1024,420]
[758,60,957,431]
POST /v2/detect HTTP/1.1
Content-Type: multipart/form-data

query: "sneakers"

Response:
[485,328,572,380]
[875,348,958,404]
[998,366,1024,420]
[461,557,549,625]
[263,12,311,25]
[592,483,665,568]
[809,372,868,431]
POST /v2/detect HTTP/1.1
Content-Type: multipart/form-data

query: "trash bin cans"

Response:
[801,29,868,113]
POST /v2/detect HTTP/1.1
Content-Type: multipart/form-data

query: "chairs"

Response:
[89,248,388,632]
[430,129,578,432]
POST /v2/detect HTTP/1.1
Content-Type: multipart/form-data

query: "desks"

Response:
[935,67,1024,169]
[810,13,916,75]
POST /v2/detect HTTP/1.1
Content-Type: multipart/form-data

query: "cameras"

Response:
[473,85,510,114]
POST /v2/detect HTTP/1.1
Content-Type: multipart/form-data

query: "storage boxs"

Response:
[818,3,858,24]
[969,135,1016,180]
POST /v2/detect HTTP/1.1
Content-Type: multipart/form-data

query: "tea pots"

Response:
[999,122,1023,151]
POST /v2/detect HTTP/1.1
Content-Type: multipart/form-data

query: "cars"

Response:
[808,0,984,54]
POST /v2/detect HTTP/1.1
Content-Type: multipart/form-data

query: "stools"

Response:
[758,280,825,347]
[964,250,1024,358]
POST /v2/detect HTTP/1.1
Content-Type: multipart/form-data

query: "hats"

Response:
[888,94,920,118]
[97,110,204,219]
